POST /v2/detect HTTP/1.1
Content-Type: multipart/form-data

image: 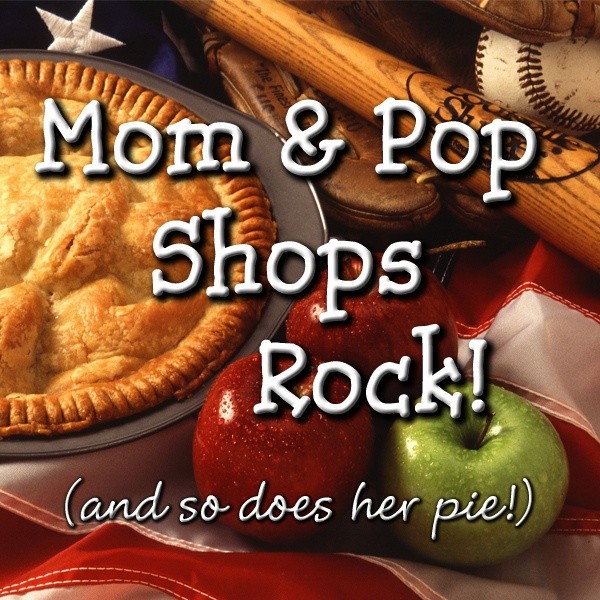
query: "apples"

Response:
[286,251,458,410]
[192,349,376,547]
[376,382,570,571]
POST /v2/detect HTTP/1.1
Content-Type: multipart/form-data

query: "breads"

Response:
[1,59,278,435]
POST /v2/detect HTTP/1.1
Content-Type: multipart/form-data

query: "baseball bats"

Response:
[179,0,600,273]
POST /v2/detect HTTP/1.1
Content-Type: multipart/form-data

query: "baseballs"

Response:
[474,24,599,138]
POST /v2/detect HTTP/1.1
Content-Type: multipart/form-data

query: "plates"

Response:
[2,44,336,461]
